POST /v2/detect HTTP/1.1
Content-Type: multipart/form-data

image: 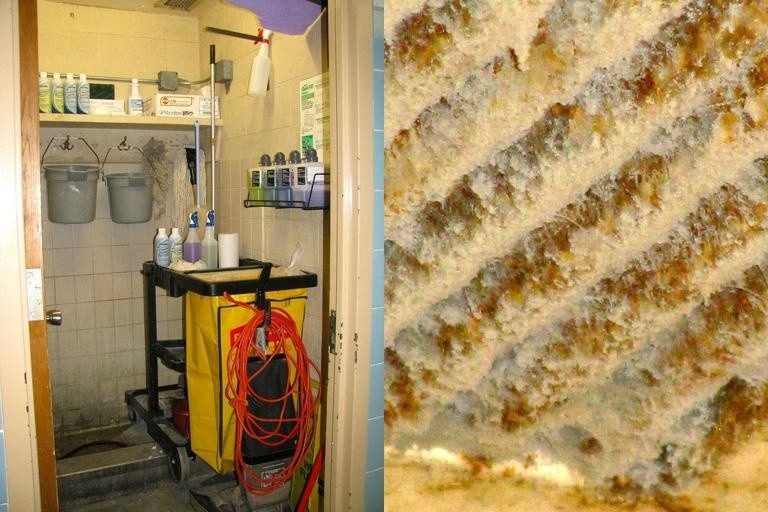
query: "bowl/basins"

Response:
[170,400,189,439]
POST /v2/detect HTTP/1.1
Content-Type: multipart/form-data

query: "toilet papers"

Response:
[217,232,241,270]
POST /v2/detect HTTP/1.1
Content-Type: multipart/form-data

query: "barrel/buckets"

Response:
[41,137,102,225]
[100,147,156,224]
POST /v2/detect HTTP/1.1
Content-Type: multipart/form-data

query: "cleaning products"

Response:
[245,148,327,209]
[183,210,202,262]
[39,71,90,114]
[153,227,170,265]
[247,27,274,96]
[201,209,217,270]
[169,227,183,263]
[128,78,143,115]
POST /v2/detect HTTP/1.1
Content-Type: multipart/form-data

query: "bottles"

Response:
[129,79,145,118]
[37,72,89,114]
[154,228,183,269]
